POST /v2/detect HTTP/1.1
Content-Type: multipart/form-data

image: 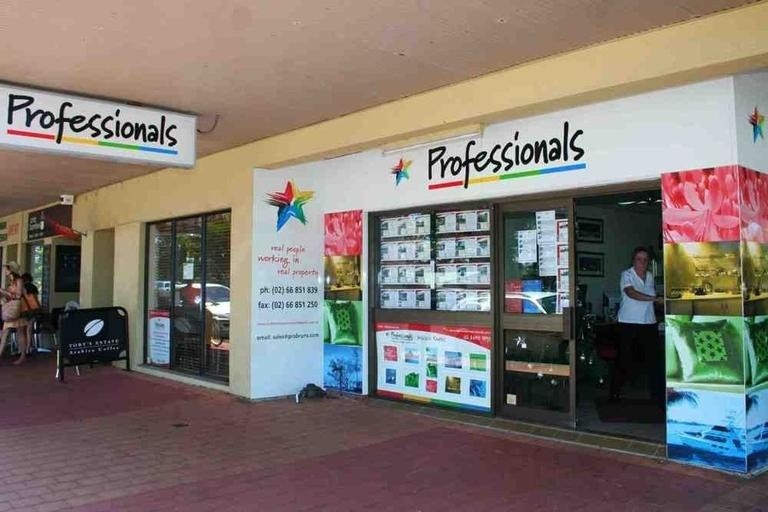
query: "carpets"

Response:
[594,396,664,424]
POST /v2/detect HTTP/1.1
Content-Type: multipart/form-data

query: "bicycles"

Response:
[210,319,223,346]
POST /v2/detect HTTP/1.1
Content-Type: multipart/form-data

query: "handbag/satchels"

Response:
[1,298,23,323]
[26,308,46,322]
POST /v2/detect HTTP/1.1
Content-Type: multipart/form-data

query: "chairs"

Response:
[504,322,620,416]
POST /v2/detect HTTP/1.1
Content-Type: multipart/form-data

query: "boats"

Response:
[678,430,768,462]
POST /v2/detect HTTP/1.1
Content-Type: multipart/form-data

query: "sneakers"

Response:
[13,352,33,365]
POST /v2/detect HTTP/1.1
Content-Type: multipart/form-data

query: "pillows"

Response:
[665,315,768,386]
[325,301,359,345]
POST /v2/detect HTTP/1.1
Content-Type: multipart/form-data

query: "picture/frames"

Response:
[576,216,605,244]
[577,250,605,278]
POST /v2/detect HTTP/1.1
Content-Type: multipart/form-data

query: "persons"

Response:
[0,261,28,367]
[15,272,40,358]
[607,246,664,406]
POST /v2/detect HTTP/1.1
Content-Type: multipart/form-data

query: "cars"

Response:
[175,284,230,338]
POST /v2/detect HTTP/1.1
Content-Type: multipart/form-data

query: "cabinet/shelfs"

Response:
[324,284,361,301]
[663,295,768,314]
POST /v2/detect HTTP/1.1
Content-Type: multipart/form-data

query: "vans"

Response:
[156,281,170,291]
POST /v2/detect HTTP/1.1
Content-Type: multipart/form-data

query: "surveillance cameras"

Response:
[59,194,74,205]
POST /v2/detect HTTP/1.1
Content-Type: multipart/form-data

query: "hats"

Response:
[2,262,24,278]
